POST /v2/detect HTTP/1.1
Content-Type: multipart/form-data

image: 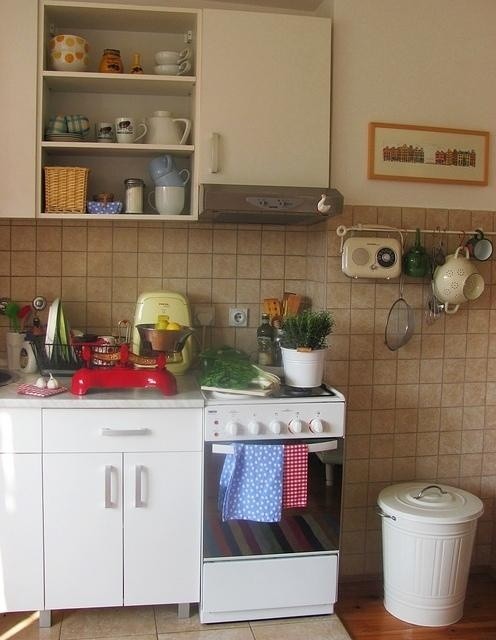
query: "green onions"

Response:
[198,346,280,384]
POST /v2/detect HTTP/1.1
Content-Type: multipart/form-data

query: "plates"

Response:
[45,132,84,142]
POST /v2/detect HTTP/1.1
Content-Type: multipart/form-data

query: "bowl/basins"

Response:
[49,34,90,71]
[134,320,194,351]
[199,351,251,375]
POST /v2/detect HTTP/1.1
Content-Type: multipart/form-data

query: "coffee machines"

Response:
[129,291,195,376]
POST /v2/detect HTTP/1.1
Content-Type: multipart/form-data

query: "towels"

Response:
[216,442,286,523]
[281,443,310,510]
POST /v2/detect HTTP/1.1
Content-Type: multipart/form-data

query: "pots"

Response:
[431,246,485,317]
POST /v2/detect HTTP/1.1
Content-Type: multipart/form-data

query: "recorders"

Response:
[341,236,403,279]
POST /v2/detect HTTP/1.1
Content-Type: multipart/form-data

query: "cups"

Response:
[95,120,114,142]
[466,227,494,261]
[154,48,194,75]
[148,185,186,215]
[147,153,175,181]
[114,117,149,143]
[157,168,191,187]
[93,336,118,366]
[6,331,26,373]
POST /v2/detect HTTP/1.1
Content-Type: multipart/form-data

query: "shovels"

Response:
[262,292,301,329]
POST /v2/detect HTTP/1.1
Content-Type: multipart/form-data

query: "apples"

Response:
[167,323,180,330]
[155,320,170,330]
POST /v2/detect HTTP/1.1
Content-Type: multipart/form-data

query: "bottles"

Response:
[406,246,429,278]
[30,318,45,336]
[127,53,145,74]
[99,48,122,73]
[123,177,145,214]
[257,313,275,367]
[17,335,38,373]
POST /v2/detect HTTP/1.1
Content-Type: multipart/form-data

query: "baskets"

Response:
[30,334,126,377]
[45,166,91,214]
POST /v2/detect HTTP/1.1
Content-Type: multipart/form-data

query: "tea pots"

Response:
[143,110,192,144]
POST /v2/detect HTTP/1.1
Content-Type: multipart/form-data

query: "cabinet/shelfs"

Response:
[43,408,198,608]
[198,8,332,187]
[40,5,194,219]
[0,403,43,617]
[1,0,38,222]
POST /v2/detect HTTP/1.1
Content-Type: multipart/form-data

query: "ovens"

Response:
[202,437,341,629]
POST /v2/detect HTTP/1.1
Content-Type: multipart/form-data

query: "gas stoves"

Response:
[196,372,345,438]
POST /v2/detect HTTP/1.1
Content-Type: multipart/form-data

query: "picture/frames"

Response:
[367,120,491,187]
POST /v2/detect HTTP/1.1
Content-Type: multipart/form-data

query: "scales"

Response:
[69,319,194,396]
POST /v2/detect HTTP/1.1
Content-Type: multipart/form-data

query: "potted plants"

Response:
[276,309,341,388]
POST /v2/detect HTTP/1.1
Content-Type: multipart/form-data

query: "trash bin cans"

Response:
[376,482,485,627]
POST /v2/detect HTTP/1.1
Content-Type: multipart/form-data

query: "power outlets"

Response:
[193,305,216,328]
[227,307,248,327]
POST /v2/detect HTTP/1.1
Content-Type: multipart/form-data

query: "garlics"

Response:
[47,372,59,389]
[34,377,46,390]
